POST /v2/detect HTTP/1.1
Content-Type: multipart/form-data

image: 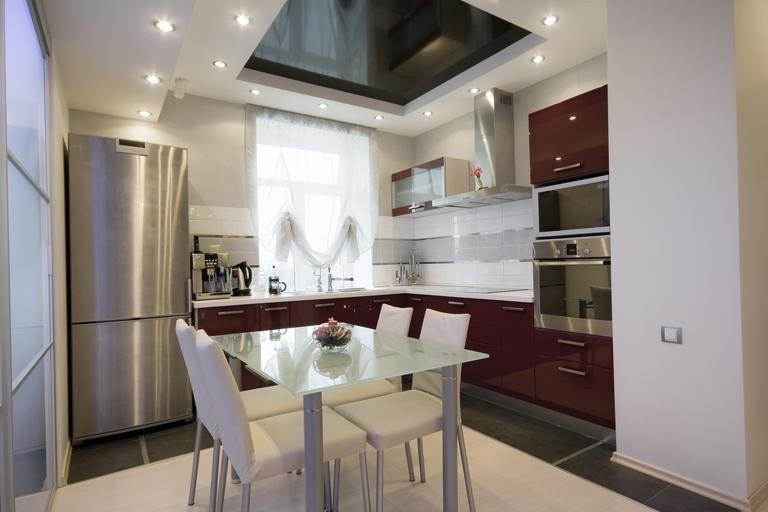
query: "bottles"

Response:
[312,323,354,352]
[272,266,277,277]
[393,260,409,285]
[272,342,278,351]
[313,350,353,379]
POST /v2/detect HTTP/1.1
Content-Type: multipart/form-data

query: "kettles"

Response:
[233,333,254,358]
[231,261,252,296]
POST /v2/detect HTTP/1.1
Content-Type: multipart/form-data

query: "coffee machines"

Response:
[191,251,231,300]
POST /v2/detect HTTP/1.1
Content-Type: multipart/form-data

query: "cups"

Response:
[268,276,287,295]
[269,329,288,342]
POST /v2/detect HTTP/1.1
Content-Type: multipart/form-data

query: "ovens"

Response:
[532,259,612,335]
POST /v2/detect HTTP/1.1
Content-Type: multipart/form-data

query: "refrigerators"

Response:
[65,130,193,445]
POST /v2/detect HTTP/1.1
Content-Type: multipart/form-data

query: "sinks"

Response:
[338,287,375,294]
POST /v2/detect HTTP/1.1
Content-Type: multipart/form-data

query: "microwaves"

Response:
[533,176,609,236]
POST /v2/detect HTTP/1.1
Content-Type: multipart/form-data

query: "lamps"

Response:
[172,76,187,100]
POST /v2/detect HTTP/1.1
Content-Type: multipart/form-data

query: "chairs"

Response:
[175,303,490,511]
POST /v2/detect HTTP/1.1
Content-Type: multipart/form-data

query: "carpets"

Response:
[49,424,659,512]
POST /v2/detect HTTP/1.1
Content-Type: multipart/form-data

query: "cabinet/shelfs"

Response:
[352,293,402,329]
[289,297,352,330]
[526,82,609,186]
[390,155,469,220]
[402,293,436,340]
[434,293,617,446]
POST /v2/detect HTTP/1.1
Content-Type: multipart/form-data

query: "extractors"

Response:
[432,90,531,210]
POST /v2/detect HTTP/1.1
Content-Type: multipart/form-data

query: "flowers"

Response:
[470,166,485,189]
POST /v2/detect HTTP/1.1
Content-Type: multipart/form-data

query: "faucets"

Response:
[327,267,354,291]
